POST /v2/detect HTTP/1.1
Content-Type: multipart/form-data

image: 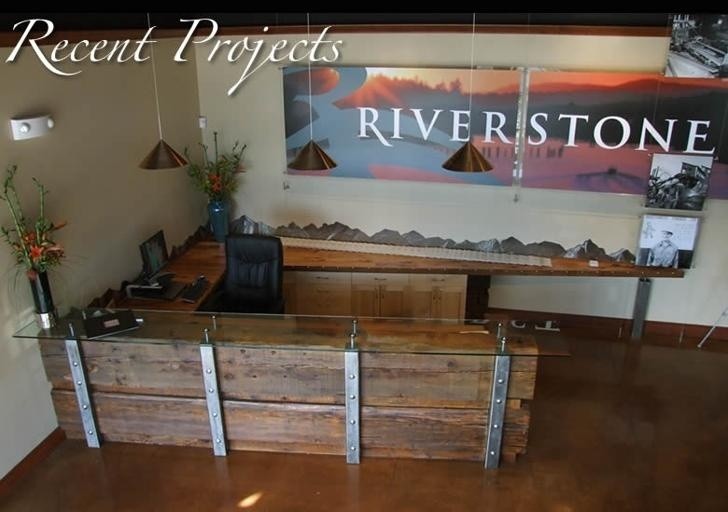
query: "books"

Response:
[82,308,140,341]
[635,214,700,271]
[643,151,713,210]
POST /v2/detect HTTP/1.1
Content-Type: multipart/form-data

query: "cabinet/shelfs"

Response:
[352,272,409,319]
[296,271,351,315]
[410,273,468,320]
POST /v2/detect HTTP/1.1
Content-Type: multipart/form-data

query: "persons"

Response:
[647,225,678,268]
[648,164,710,211]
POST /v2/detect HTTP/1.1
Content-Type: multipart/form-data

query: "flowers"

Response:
[0,165,66,272]
[182,131,246,195]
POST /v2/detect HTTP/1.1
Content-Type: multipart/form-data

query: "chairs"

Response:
[200,236,285,314]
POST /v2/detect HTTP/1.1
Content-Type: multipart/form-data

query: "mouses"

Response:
[198,275,208,281]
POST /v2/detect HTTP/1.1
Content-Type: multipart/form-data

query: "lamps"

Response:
[139,14,188,169]
[287,14,337,170]
[441,13,493,173]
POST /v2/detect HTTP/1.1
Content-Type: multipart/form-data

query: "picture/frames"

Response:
[139,229,169,272]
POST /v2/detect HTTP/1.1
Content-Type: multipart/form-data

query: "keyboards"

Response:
[181,281,209,304]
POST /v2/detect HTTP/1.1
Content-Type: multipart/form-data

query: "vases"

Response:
[208,201,232,242]
[27,268,58,329]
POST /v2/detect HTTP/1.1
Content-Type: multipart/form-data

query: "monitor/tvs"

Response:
[138,228,176,287]
